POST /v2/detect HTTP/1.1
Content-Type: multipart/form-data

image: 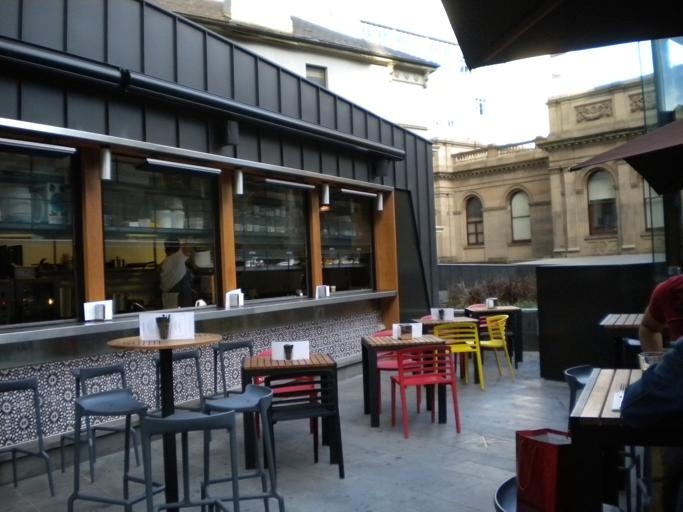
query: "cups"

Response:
[156,317,169,339]
[331,285,336,293]
[284,344,293,360]
[438,310,444,320]
[638,351,666,377]
[493,298,498,307]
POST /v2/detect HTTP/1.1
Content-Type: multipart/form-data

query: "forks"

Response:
[619,384,625,391]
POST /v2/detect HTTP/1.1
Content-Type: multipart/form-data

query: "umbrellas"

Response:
[569,105,683,196]
[441,0,683,72]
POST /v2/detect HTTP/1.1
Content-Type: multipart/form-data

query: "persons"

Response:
[620,336,683,430]
[639,275,683,356]
[158,236,193,309]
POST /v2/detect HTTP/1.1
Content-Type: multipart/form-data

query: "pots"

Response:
[12,264,40,280]
[108,255,125,269]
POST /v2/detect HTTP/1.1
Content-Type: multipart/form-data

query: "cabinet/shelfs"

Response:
[0,169,364,240]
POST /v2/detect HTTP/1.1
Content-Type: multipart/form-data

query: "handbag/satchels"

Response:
[514,427,604,511]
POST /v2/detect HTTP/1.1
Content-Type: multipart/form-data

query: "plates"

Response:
[192,250,214,268]
[125,208,205,230]
[245,259,302,267]
[232,204,289,234]
